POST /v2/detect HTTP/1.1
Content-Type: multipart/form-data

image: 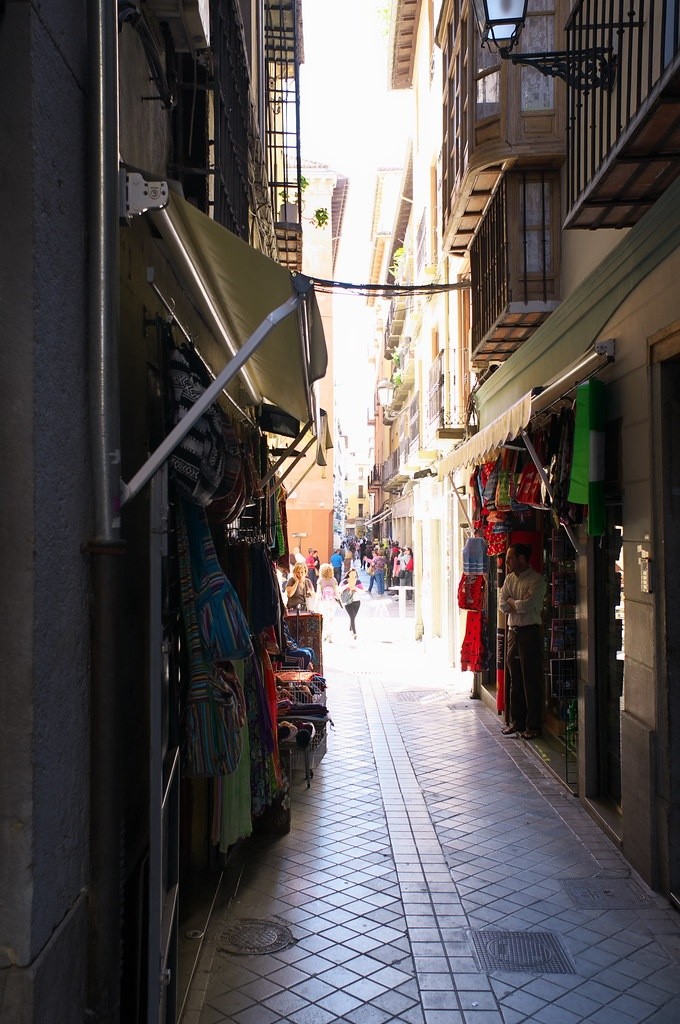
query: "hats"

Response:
[393,548,399,553]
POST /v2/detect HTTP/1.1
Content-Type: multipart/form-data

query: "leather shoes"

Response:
[521,728,542,740]
[502,721,521,734]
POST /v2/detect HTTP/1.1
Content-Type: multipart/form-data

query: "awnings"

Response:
[434,344,615,549]
[125,190,334,502]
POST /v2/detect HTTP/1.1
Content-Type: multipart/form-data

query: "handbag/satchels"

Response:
[315,567,319,576]
[340,580,353,603]
[147,343,264,524]
[181,528,254,778]
[480,430,552,512]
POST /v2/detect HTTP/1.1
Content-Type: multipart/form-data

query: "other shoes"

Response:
[353,634,357,640]
[389,592,395,595]
[385,589,389,590]
[365,590,371,594]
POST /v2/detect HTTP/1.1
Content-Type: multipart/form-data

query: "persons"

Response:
[340,535,413,600]
[497,543,548,739]
[283,605,315,668]
[339,568,364,639]
[330,549,343,584]
[276,563,286,592]
[317,563,340,643]
[287,563,314,611]
[289,546,320,592]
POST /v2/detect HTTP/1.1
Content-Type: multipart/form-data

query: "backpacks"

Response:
[321,577,336,601]
[404,555,413,570]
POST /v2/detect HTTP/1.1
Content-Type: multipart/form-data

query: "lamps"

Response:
[472,0,615,90]
[253,402,300,439]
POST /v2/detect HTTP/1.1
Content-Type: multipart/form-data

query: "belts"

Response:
[509,626,540,633]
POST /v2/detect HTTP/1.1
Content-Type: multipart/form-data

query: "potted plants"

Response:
[278,176,330,230]
